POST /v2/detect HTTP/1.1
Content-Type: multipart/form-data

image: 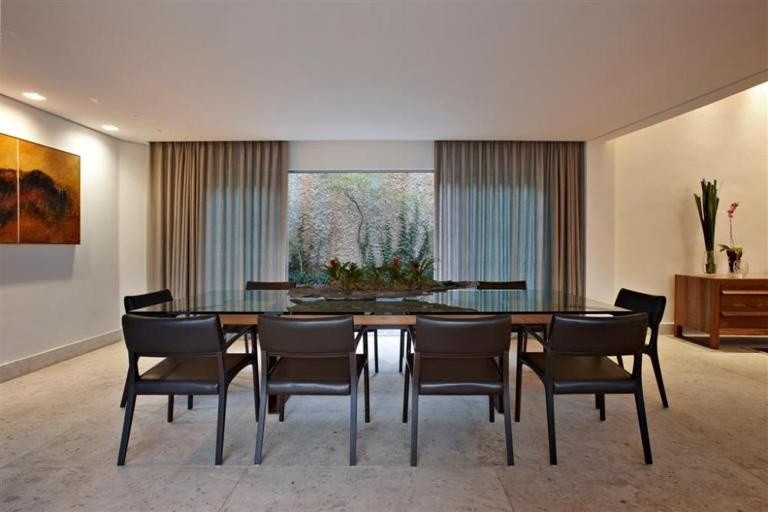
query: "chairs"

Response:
[514,311,655,466]
[116,314,261,468]
[258,313,370,467]
[119,288,194,410]
[400,315,515,468]
[594,288,669,409]
[223,281,296,354]
[476,280,529,290]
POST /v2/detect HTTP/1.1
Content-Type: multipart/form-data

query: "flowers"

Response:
[320,256,440,288]
[718,202,743,255]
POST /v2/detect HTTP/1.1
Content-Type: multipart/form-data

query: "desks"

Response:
[129,290,632,423]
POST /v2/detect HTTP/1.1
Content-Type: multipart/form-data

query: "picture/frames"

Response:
[0,132,81,245]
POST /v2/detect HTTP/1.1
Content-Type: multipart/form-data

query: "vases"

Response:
[726,249,742,272]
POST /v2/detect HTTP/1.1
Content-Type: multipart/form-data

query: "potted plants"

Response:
[693,178,719,274]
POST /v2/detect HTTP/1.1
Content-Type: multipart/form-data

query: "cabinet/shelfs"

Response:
[674,274,768,350]
